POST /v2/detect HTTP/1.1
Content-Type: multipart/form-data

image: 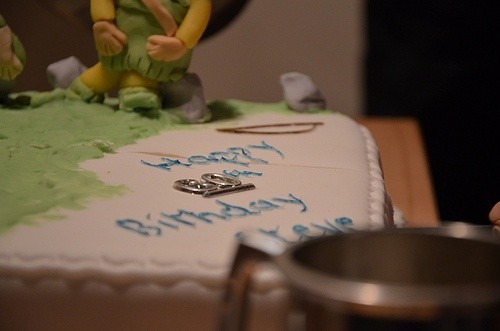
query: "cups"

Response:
[276,227,500,331]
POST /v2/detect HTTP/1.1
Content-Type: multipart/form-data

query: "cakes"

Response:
[0,1,397,331]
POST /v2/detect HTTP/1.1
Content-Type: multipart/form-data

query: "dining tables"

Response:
[352,110,441,228]
